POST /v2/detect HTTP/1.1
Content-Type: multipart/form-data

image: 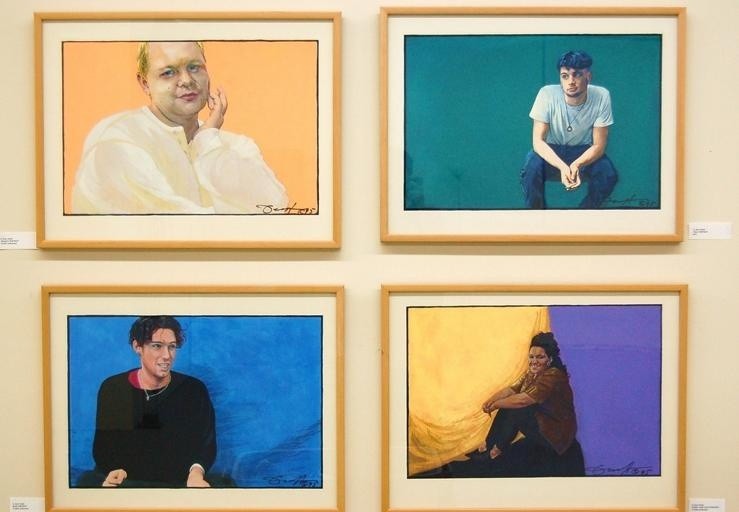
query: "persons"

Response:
[71,40,288,213]
[520,51,618,209]
[76,316,234,489]
[466,331,577,467]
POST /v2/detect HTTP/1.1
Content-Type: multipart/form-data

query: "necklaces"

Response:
[564,96,588,132]
[139,375,172,402]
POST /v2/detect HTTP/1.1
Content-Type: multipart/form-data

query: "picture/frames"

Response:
[379,7,686,244]
[381,283,689,512]
[42,284,344,512]
[34,11,343,249]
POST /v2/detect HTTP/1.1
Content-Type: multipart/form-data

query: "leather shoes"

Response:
[452,448,506,470]
